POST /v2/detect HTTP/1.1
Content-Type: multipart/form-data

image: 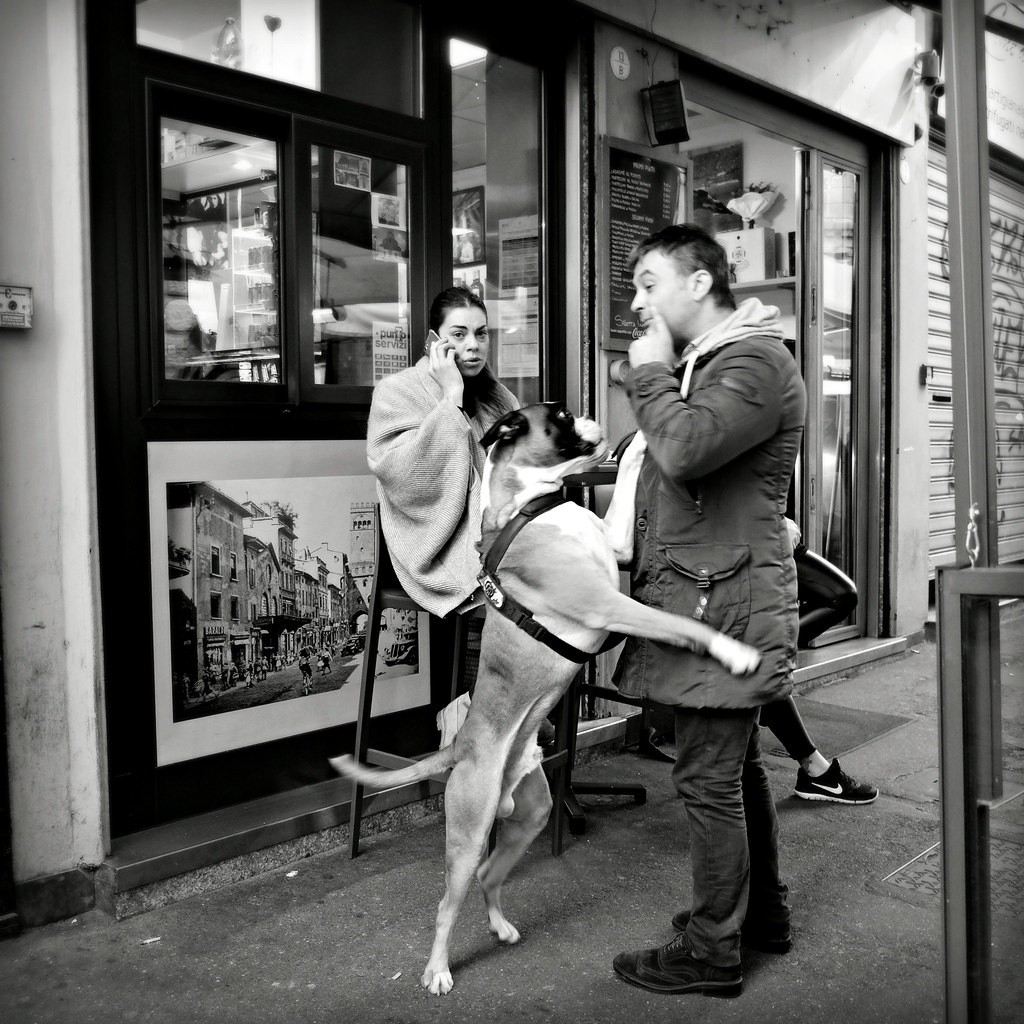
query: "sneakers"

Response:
[795,757,879,805]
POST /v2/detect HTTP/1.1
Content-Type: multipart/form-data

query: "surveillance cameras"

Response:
[921,53,945,98]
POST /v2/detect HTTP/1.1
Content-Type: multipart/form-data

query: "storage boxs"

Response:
[713,226,775,282]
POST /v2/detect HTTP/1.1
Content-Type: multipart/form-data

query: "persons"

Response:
[366,284,531,756]
[611,221,812,997]
[180,642,337,705]
[757,515,880,808]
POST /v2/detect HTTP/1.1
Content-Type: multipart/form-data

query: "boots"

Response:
[436,690,472,751]
[538,717,555,745]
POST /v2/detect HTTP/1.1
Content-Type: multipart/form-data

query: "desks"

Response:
[551,460,647,834]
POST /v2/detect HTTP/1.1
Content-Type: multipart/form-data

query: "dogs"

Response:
[330,401,764,997]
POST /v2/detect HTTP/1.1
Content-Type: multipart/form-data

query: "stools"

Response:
[345,503,578,859]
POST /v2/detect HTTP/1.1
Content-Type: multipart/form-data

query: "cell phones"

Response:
[424,329,440,356]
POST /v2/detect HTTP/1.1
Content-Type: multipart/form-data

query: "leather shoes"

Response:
[672,907,792,955]
[612,932,744,998]
